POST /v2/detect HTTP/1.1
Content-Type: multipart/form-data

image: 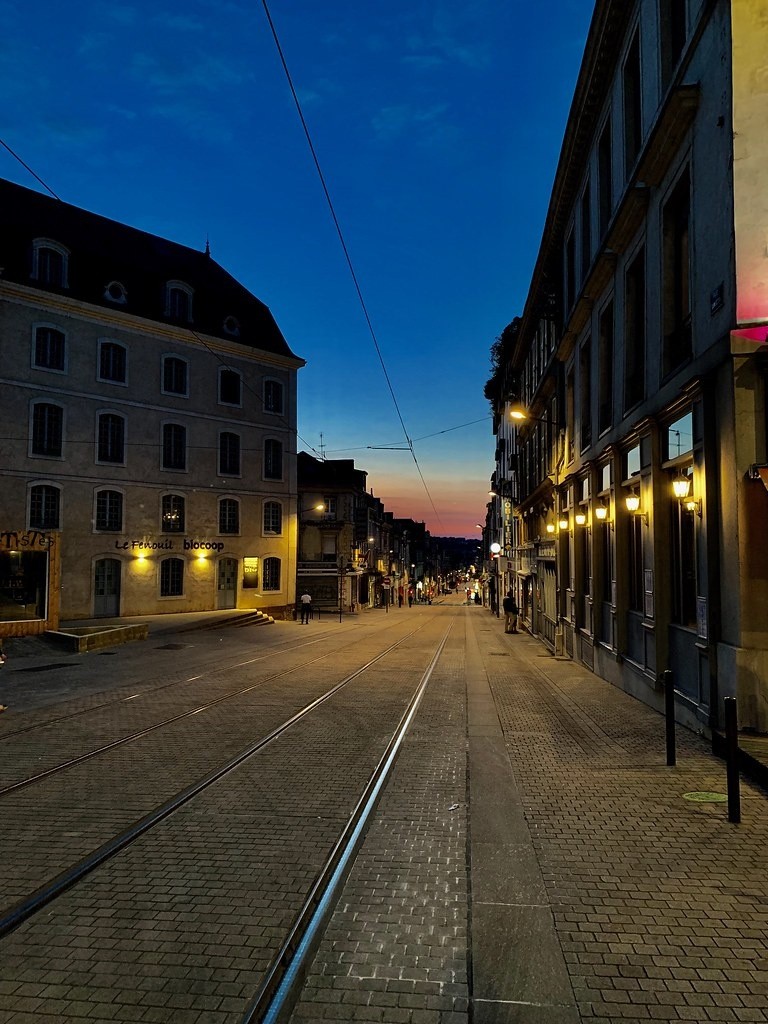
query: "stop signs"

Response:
[382,578,390,584]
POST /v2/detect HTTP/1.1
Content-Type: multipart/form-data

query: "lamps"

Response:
[559,515,573,538]
[490,543,503,552]
[546,518,560,540]
[595,500,614,532]
[476,524,498,532]
[625,486,649,527]
[291,504,324,515]
[575,508,591,535]
[488,490,526,501]
[672,473,703,519]
[510,409,566,429]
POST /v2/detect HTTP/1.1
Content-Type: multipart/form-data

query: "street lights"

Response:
[491,543,500,619]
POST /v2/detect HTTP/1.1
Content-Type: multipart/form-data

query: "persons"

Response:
[408,594,413,608]
[456,587,459,594]
[301,590,311,624]
[418,592,431,605]
[444,590,447,596]
[503,591,523,634]
[398,593,403,608]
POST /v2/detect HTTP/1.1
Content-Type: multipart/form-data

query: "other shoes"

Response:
[0,704,8,713]
